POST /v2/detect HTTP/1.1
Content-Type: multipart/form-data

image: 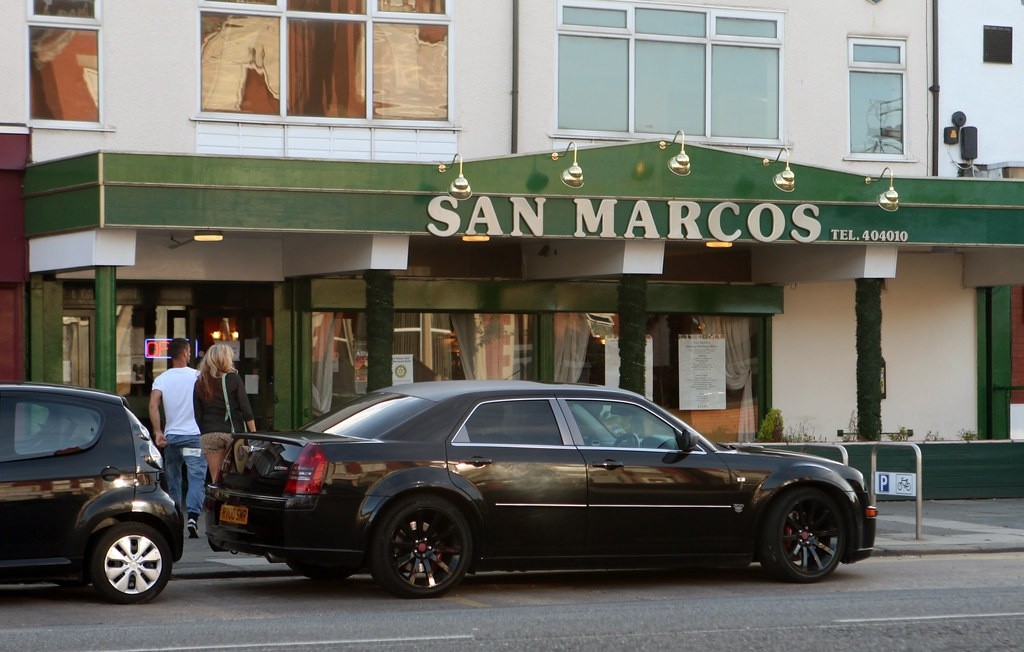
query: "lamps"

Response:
[763,146,795,193]
[865,167,899,212]
[659,130,690,176]
[439,153,472,201]
[194,230,223,241]
[552,141,584,189]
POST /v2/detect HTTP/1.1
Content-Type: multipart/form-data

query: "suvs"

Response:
[0,381,187,606]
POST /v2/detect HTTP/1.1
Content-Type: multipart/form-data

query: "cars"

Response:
[201,378,878,600]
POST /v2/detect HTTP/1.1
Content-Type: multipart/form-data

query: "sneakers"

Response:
[187,518,200,538]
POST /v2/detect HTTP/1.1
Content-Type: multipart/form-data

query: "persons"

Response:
[193,343,256,485]
[149,337,208,538]
